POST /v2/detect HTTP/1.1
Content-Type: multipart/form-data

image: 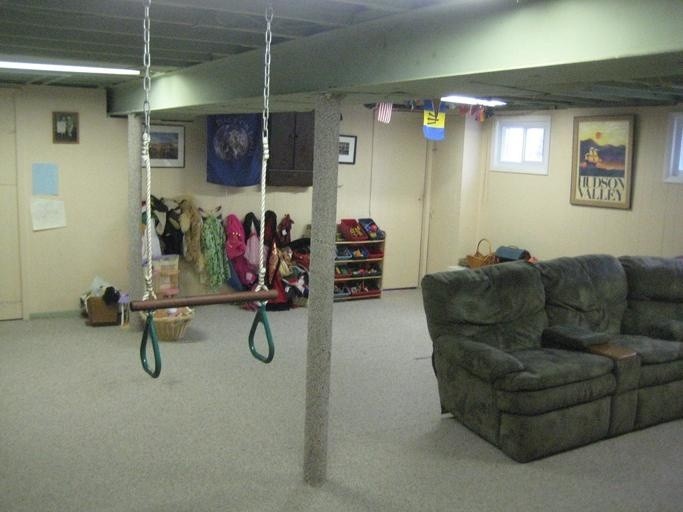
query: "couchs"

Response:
[421,253,683,464]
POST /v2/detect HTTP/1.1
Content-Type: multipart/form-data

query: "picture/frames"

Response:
[569,114,635,210]
[338,134,357,164]
[142,122,185,168]
[52,111,80,144]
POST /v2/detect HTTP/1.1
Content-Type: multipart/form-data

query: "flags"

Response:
[421,101,449,142]
[377,101,393,126]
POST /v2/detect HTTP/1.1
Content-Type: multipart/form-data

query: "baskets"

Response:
[139,304,195,342]
[467,238,494,269]
[85,296,118,324]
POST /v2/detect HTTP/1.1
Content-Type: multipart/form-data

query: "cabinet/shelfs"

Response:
[266,111,314,187]
[334,230,385,301]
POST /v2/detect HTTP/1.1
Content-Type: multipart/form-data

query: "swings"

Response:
[130,0,275,378]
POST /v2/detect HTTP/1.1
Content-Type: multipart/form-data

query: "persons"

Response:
[65,114,74,138]
[55,114,66,139]
